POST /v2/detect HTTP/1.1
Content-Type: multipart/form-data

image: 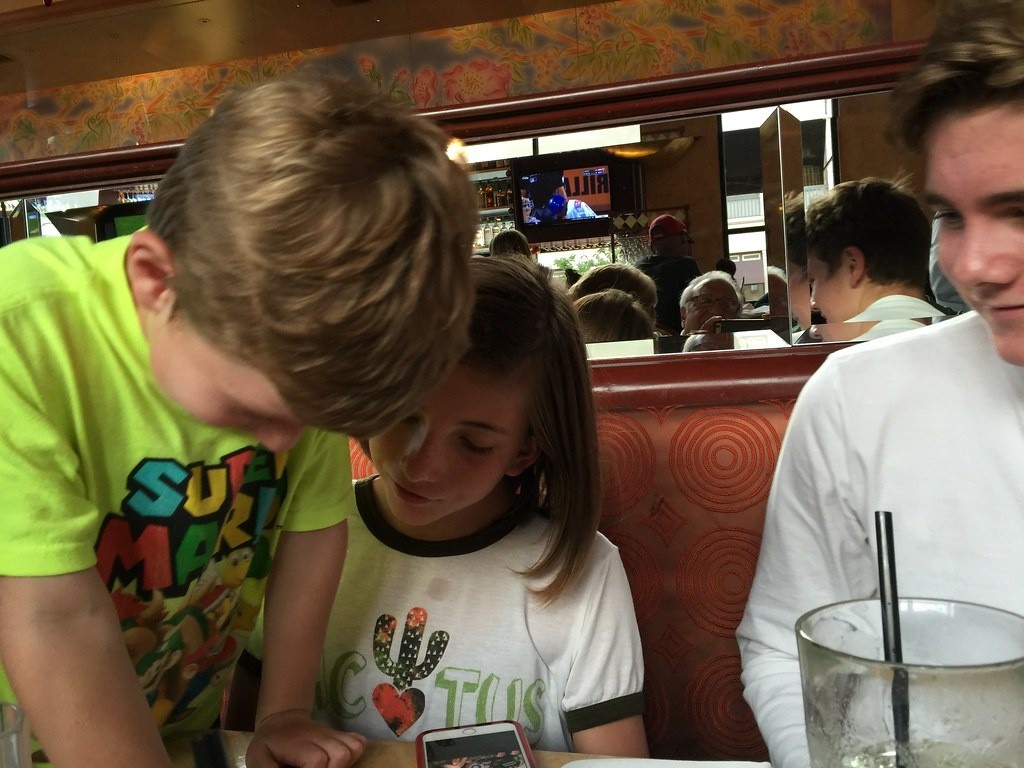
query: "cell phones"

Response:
[715,318,765,331]
[416,720,536,768]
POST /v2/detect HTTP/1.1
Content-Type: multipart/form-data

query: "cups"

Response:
[795,597,1024,768]
[0,702,34,768]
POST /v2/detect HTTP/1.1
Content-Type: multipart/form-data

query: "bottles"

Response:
[475,179,515,246]
[119,184,159,202]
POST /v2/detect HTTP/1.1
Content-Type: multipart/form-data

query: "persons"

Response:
[0,78,478,768]
[717,259,745,294]
[548,195,597,219]
[767,266,789,317]
[522,169,567,224]
[489,230,533,259]
[566,262,657,313]
[633,214,703,331]
[679,272,741,334]
[754,292,769,308]
[231,257,647,757]
[572,291,653,343]
[734,19,1023,768]
[807,176,946,320]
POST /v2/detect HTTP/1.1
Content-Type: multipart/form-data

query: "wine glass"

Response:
[616,237,651,262]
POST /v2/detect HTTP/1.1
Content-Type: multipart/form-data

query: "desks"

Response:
[580,329,794,359]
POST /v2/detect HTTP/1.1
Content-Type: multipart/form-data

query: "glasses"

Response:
[685,295,742,310]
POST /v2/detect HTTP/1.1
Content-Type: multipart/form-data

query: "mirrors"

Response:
[0,41,972,413]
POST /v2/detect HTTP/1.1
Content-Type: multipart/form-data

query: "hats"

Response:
[649,214,696,246]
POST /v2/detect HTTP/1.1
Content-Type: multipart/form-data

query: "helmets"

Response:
[534,193,566,222]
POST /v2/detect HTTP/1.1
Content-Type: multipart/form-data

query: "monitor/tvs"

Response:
[511,148,618,232]
